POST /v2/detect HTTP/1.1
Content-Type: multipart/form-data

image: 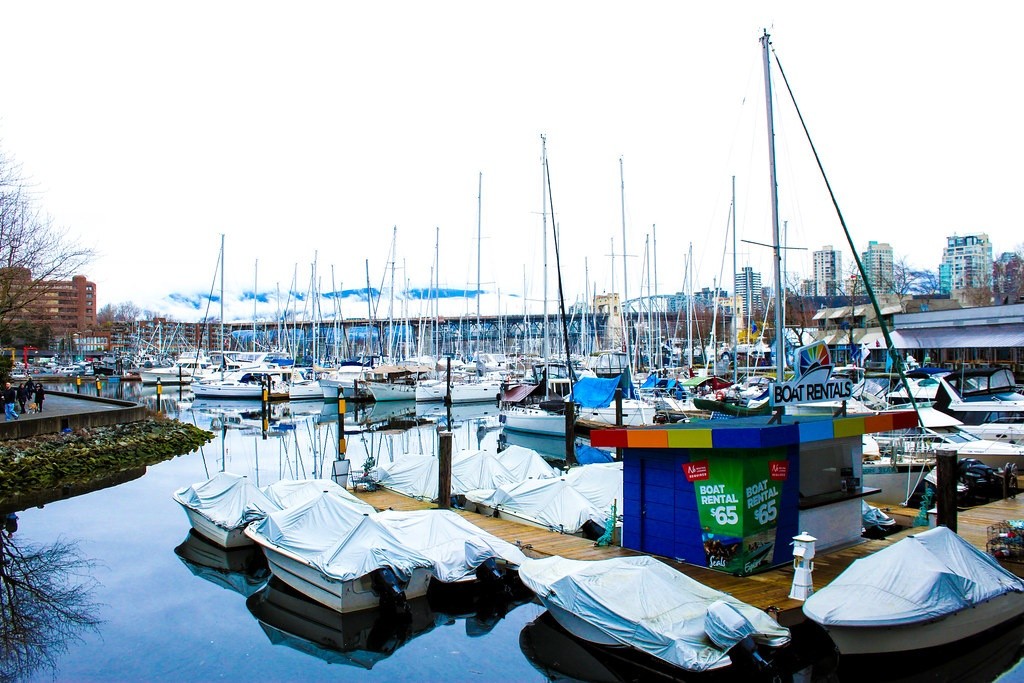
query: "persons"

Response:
[0,378,45,421]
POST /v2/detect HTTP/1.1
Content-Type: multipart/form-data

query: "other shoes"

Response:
[19,411,26,414]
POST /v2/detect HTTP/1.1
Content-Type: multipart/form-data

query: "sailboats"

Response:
[188,397,289,472]
[120,16,1024,503]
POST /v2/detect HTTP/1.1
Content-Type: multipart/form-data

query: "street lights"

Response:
[77,331,82,360]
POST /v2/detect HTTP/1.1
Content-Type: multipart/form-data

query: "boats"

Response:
[801,524,1023,666]
[245,575,534,673]
[173,474,274,550]
[10,357,115,377]
[171,528,269,598]
[371,508,524,620]
[793,614,1024,683]
[264,479,376,517]
[519,608,813,683]
[243,491,433,619]
[515,551,791,682]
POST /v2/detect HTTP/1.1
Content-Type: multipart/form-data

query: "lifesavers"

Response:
[716,391,724,401]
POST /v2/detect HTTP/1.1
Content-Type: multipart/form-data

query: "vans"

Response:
[61,367,75,373]
[11,372,26,377]
